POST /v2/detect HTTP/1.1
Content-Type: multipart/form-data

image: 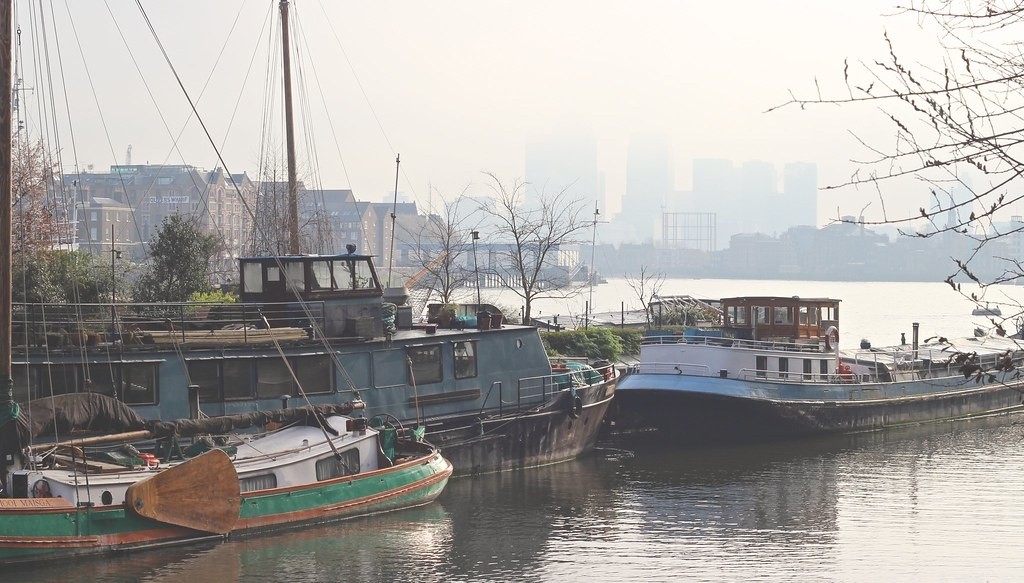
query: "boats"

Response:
[615,296,1024,438]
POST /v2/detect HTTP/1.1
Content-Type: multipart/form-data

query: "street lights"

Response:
[469,227,480,308]
[101,224,127,344]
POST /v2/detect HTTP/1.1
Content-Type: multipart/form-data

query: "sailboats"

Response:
[0,1,454,566]
[1,2,639,481]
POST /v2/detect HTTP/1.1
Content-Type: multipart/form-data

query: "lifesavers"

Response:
[826,325,841,353]
[138,452,160,466]
[567,396,583,419]
